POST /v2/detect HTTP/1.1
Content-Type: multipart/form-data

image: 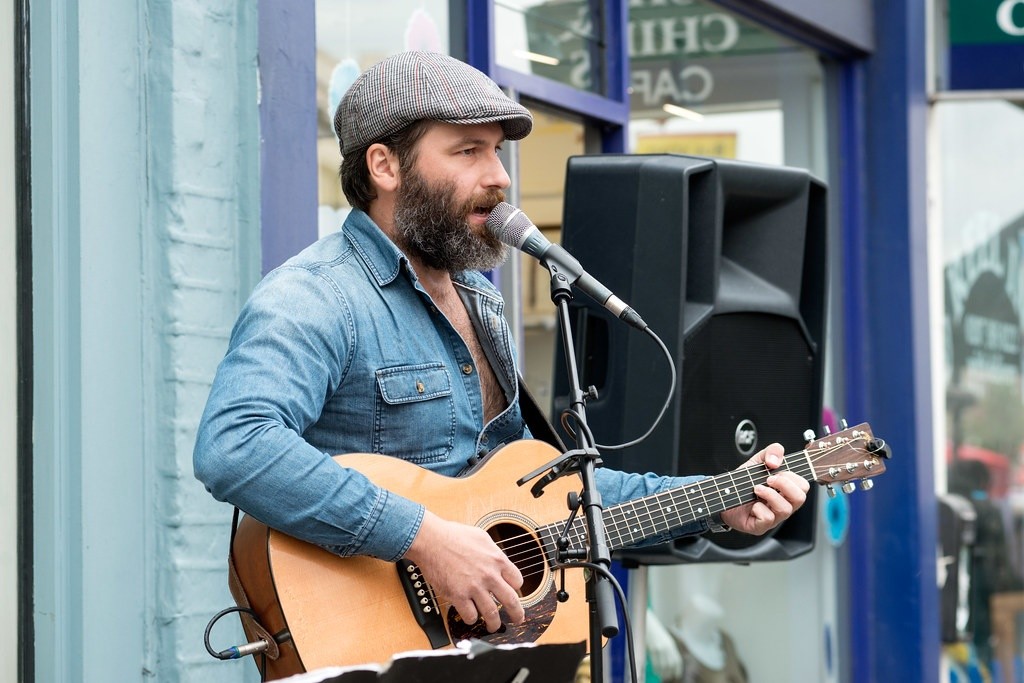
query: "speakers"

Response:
[549,156,827,564]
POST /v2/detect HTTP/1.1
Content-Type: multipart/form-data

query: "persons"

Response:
[191,50,810,683]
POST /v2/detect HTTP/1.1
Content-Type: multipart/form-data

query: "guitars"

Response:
[216,420,902,683]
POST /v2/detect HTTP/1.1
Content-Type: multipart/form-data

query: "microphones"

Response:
[485,202,649,331]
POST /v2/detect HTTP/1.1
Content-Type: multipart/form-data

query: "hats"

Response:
[333,51,532,156]
